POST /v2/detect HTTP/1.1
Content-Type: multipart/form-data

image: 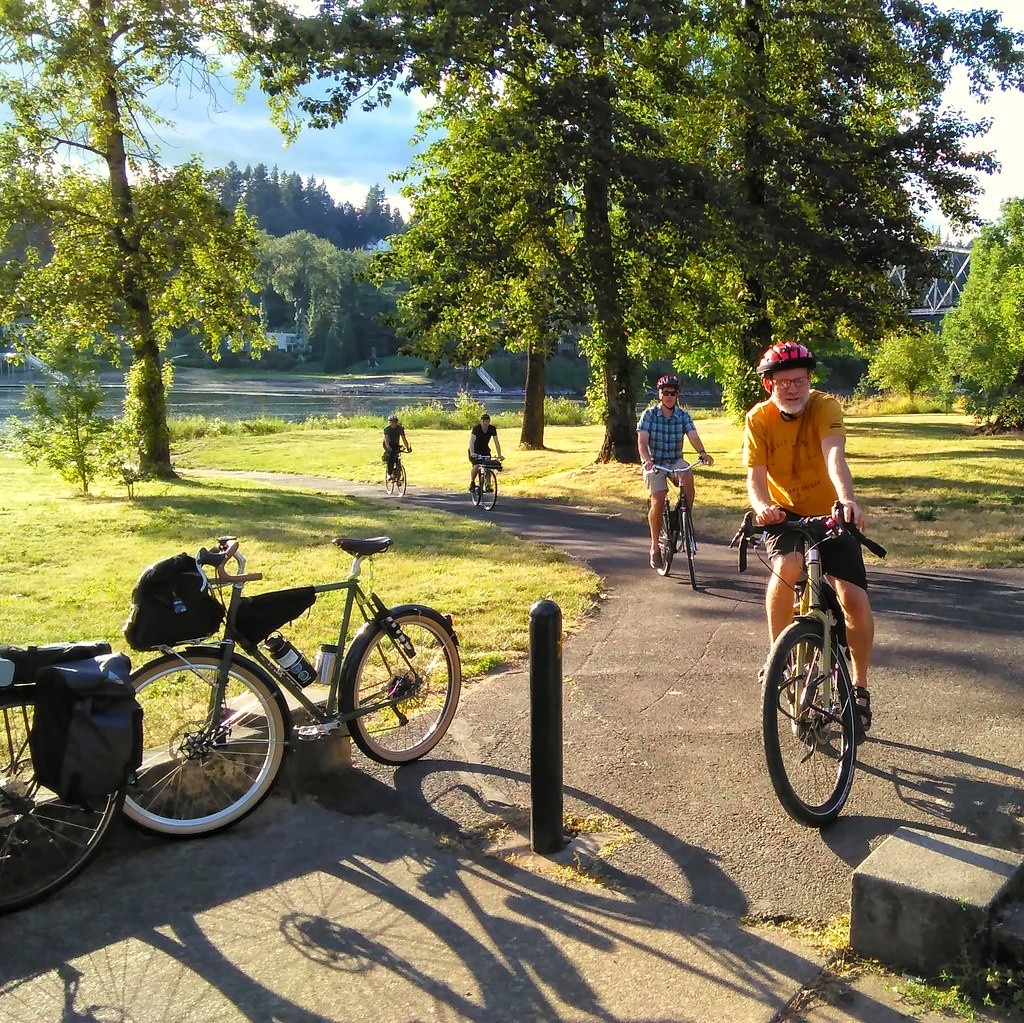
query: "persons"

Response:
[743,340,875,734]
[383,415,412,486]
[636,376,715,569]
[468,414,505,494]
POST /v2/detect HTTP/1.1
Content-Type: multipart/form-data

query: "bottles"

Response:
[262,629,317,689]
[312,644,340,686]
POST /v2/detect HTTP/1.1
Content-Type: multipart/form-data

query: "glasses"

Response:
[662,390,678,396]
[770,377,810,389]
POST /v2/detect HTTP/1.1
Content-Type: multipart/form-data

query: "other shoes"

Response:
[486,485,494,492]
[470,482,475,492]
[398,481,401,486]
[649,548,663,569]
[387,475,392,481]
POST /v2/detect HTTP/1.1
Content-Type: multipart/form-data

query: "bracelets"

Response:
[698,450,706,454]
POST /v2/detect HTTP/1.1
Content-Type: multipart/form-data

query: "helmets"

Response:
[388,415,398,421]
[657,375,679,389]
[756,342,816,374]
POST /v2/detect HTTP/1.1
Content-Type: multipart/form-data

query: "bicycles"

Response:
[384,447,412,497]
[729,500,887,828]
[0,640,145,916]
[118,534,463,841]
[644,455,708,590]
[468,454,506,512]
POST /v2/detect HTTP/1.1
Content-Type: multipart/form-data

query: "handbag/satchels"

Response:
[28,652,145,807]
[124,554,226,651]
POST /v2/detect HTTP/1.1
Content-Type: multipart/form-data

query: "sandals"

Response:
[758,655,788,683]
[853,686,872,731]
